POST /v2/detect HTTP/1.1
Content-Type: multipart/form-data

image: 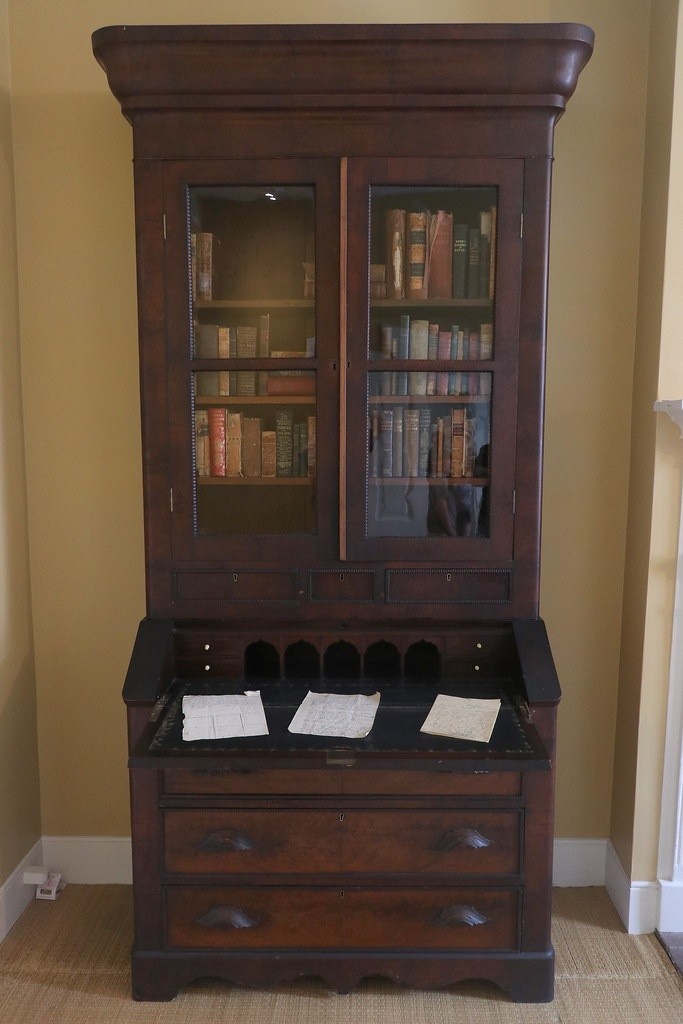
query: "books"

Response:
[186,206,495,478]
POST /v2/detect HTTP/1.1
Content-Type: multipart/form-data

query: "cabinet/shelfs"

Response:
[91,23,598,1003]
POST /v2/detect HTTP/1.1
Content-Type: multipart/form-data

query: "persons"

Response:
[433,443,490,537]
